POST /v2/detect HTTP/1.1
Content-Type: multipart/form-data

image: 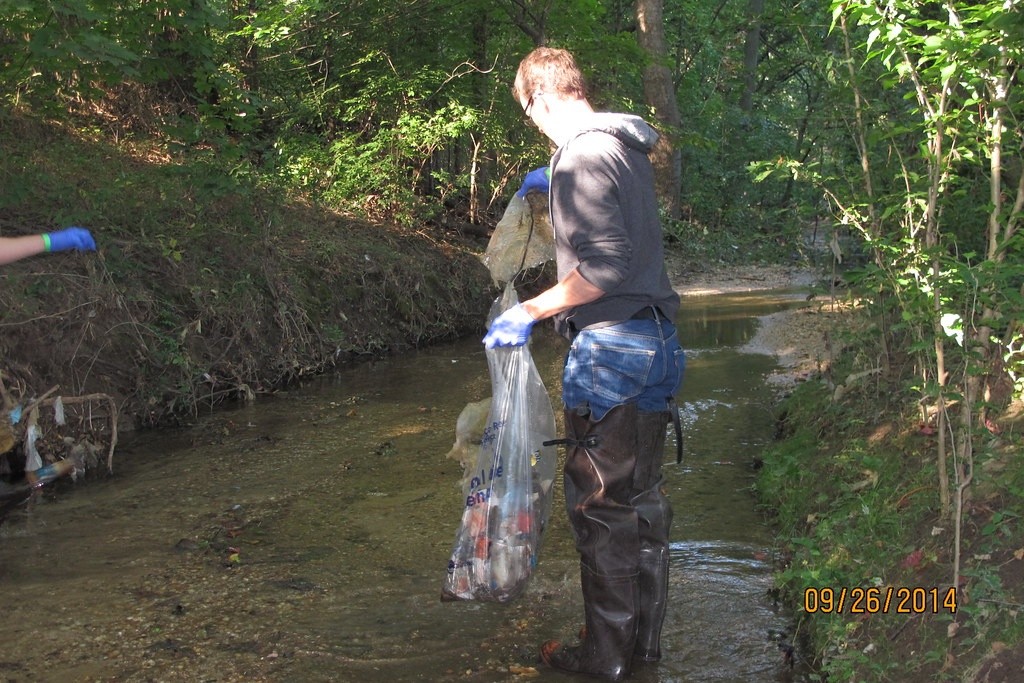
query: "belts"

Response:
[567,305,667,333]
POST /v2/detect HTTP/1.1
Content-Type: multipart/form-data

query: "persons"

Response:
[0,226,96,266]
[477,47,686,683]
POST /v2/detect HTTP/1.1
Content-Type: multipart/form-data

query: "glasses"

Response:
[519,90,544,127]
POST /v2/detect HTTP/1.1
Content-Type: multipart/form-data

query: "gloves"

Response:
[47,227,96,255]
[482,304,536,349]
[516,167,549,198]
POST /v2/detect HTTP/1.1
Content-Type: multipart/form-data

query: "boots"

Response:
[540,561,641,683]
[580,544,669,664]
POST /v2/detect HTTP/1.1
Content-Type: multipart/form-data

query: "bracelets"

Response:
[545,167,550,179]
[41,232,51,252]
